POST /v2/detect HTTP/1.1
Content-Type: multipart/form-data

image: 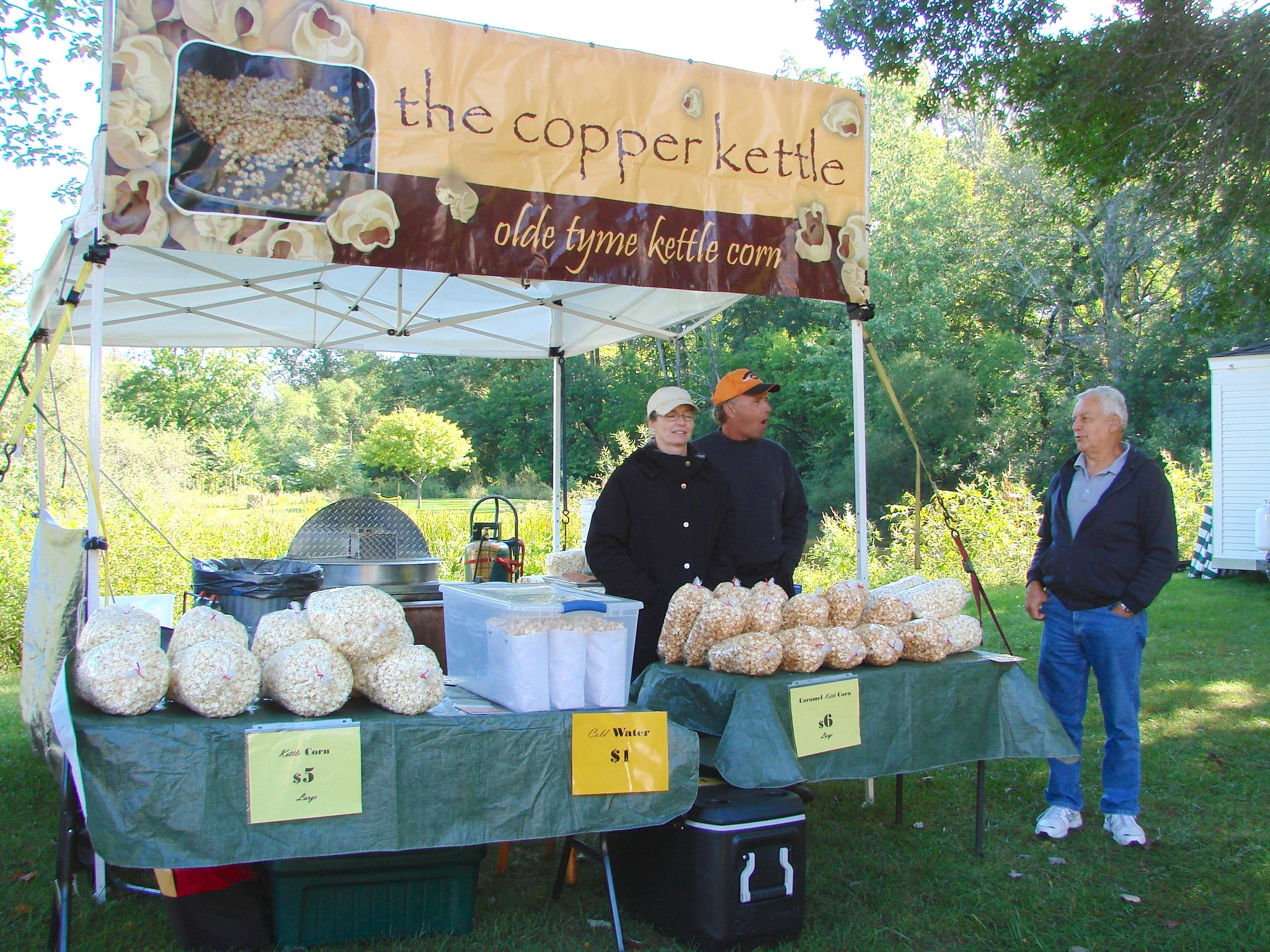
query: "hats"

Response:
[647,386,701,418]
[711,368,780,404]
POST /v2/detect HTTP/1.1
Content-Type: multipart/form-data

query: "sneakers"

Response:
[1034,805,1082,840]
[1103,814,1146,846]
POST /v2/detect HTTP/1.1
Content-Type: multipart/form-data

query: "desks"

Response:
[48,673,701,951]
[627,648,1080,852]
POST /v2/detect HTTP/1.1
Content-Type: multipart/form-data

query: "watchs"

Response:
[1119,600,1136,617]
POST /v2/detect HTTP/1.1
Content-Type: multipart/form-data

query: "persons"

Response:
[688,368,807,599]
[1025,384,1179,847]
[585,387,740,686]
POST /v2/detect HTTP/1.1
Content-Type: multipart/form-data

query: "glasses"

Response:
[649,413,697,421]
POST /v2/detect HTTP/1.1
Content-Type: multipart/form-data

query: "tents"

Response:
[21,172,874,908]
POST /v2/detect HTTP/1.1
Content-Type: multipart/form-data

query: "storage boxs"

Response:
[578,498,600,549]
[601,765,809,949]
[153,842,488,952]
[441,581,646,711]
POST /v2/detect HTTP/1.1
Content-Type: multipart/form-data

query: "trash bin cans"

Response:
[190,553,324,650]
